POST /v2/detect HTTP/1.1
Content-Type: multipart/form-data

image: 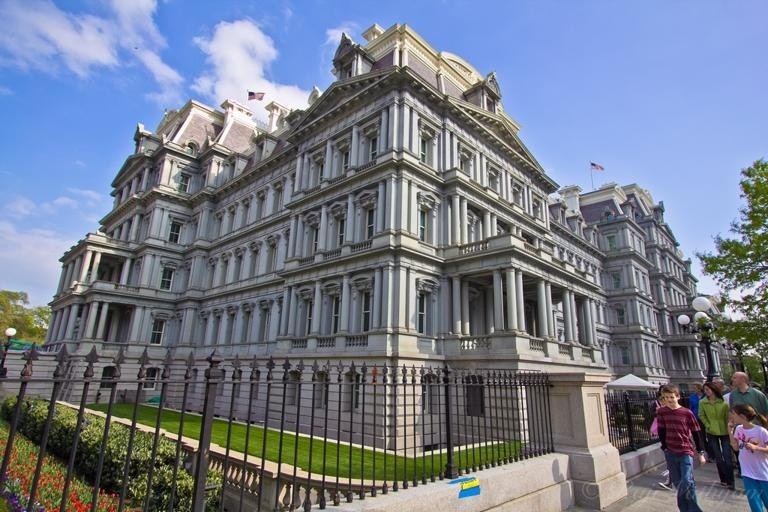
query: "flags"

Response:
[591,162,605,171]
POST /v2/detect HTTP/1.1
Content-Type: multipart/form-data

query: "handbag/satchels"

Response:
[650,418,659,435]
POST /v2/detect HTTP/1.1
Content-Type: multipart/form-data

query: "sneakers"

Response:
[659,475,673,490]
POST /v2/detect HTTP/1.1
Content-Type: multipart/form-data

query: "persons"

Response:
[649,371,767,512]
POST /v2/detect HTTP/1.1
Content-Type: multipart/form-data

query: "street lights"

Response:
[0,327,17,379]
[678,297,751,382]
